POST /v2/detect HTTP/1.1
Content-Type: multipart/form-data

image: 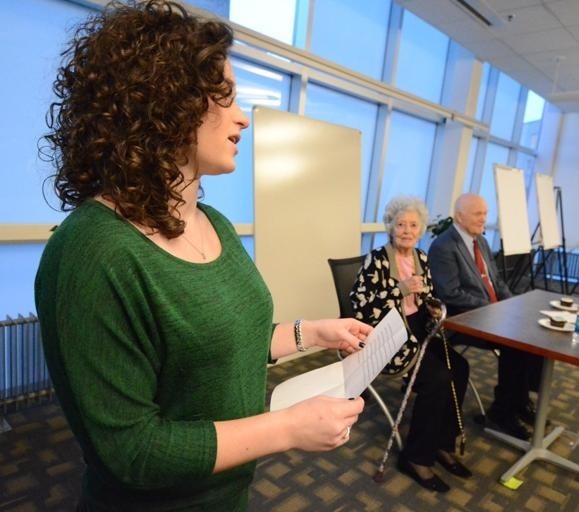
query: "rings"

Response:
[34,9,377,512]
[343,425,355,441]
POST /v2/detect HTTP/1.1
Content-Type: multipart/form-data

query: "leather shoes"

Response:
[518,397,552,427]
[485,410,530,439]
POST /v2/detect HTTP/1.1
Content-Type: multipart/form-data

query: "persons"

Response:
[348,199,473,493]
[430,194,552,441]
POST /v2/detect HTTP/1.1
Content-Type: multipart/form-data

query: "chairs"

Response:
[327,254,406,452]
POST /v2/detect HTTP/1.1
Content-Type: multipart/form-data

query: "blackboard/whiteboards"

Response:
[535,174,562,251]
[494,164,532,256]
[253,106,362,369]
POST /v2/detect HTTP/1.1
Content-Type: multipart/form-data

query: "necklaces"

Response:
[167,195,216,262]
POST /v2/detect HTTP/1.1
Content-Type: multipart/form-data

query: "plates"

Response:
[538,318,574,331]
[550,301,578,311]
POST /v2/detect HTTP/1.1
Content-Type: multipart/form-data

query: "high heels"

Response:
[396,451,450,492]
[435,448,470,478]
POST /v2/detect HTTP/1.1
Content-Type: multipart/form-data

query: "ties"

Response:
[473,238,499,304]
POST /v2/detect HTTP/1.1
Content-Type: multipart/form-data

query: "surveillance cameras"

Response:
[506,13,516,22]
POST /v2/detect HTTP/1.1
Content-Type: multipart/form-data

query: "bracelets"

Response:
[294,317,305,352]
